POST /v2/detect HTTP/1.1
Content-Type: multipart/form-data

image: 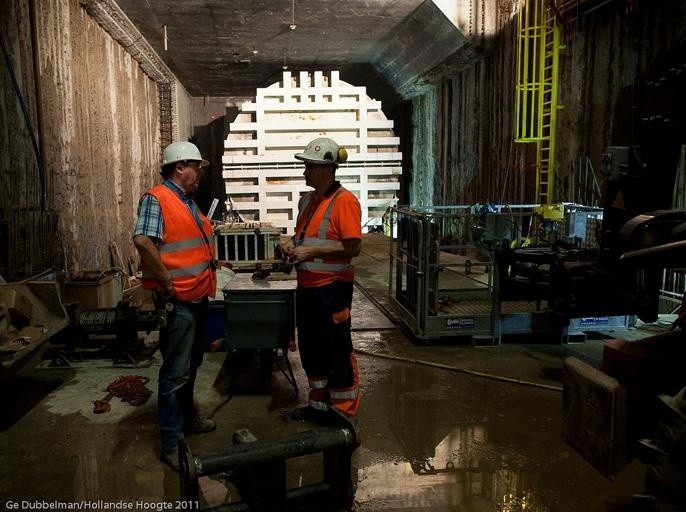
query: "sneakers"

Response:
[192,415,215,432]
[292,405,316,421]
[161,448,180,472]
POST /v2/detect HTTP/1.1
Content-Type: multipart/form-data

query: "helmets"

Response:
[162,141,211,167]
[295,138,348,165]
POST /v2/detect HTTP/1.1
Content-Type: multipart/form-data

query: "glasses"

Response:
[184,162,201,169]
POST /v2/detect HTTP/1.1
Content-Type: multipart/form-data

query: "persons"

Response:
[279,138,362,427]
[132,141,217,476]
